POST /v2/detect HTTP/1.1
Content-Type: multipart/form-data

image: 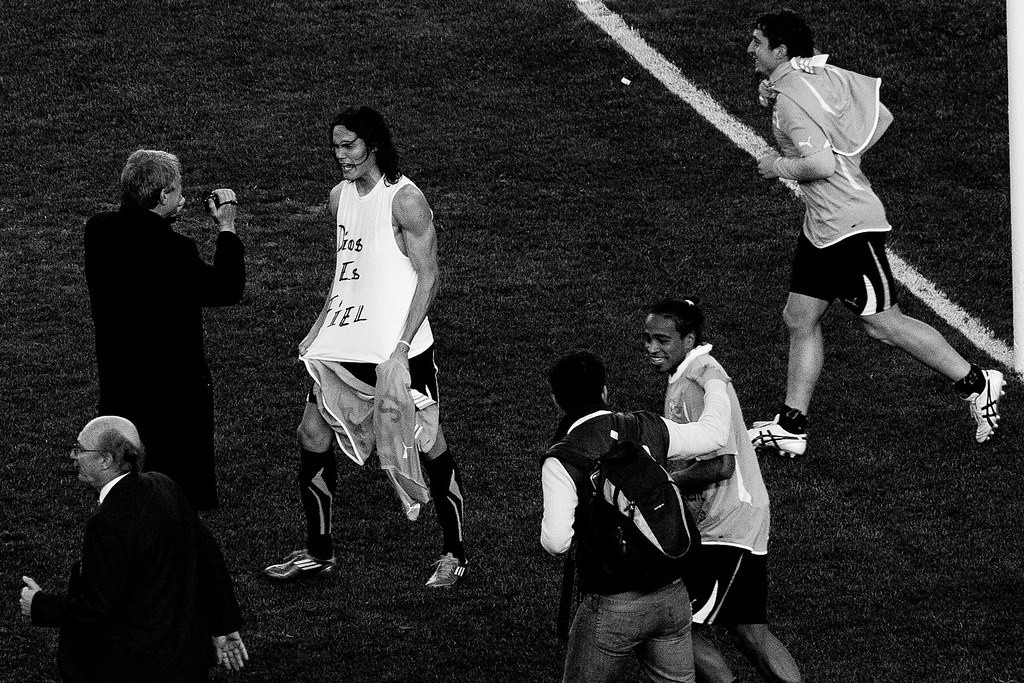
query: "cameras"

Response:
[206,195,220,214]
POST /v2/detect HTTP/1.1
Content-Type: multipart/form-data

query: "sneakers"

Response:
[423,551,468,587]
[749,415,807,458]
[960,367,1007,443]
[265,550,335,578]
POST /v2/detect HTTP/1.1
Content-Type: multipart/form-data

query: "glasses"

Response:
[71,440,104,455]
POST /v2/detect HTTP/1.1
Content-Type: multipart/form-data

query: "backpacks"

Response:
[541,413,700,597]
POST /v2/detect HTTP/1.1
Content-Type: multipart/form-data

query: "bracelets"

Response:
[396,340,411,350]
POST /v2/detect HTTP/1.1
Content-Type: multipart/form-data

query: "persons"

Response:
[644,298,803,682]
[748,6,1007,457]
[263,106,466,586]
[80,150,249,513]
[20,414,249,682]
[538,347,730,682]
